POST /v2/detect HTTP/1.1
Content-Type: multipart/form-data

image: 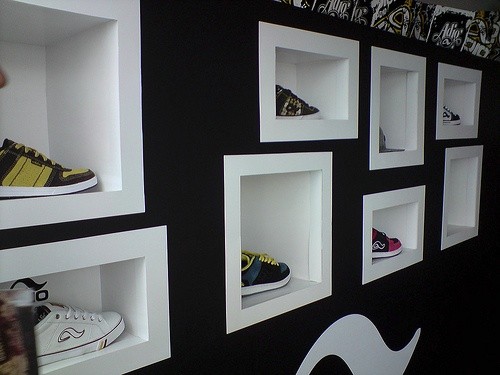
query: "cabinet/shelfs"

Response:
[0,0,500,374]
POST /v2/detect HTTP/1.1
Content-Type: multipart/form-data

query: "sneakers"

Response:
[9,277,125,366]
[442,104,462,124]
[275,84,321,119]
[0,138,98,197]
[372,228,403,257]
[241,249,291,295]
